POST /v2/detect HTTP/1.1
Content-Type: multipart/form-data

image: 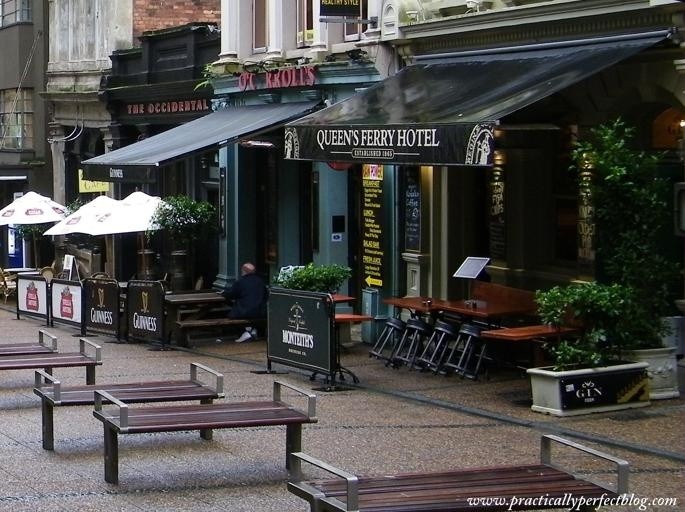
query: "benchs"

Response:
[2,329,317,487]
[277,434,631,512]
[176,318,250,347]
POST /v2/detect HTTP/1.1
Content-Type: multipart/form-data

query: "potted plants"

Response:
[525,120,683,421]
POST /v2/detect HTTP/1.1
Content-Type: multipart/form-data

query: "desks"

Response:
[164,293,224,341]
[384,289,532,320]
[481,321,578,363]
[327,291,374,384]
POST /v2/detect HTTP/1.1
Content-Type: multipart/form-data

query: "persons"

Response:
[215,262,266,343]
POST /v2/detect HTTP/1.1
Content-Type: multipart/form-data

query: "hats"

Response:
[241,263,256,274]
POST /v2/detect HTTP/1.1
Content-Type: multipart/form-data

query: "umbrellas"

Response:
[0,190,75,272]
[39,194,118,273]
[76,191,203,286]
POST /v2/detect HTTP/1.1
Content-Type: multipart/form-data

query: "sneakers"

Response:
[251,329,258,339]
[235,331,252,343]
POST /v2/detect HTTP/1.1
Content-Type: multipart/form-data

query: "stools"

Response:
[368,315,482,380]
